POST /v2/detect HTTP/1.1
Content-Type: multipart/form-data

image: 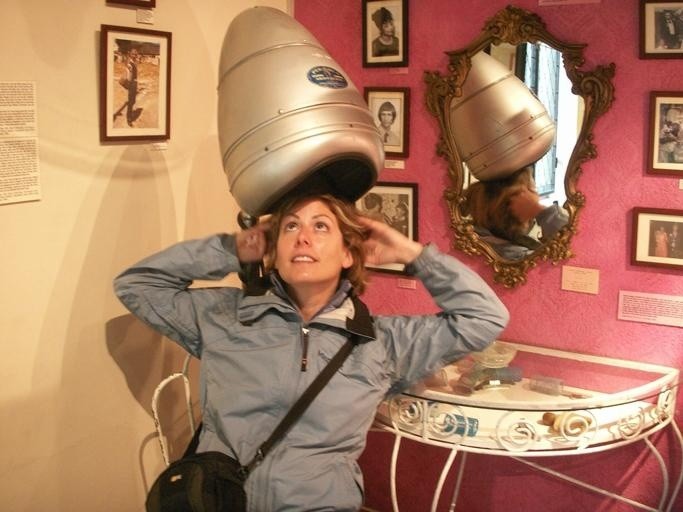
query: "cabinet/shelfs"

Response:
[334,334,682,512]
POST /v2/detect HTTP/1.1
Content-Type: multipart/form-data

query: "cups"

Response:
[434,414,479,435]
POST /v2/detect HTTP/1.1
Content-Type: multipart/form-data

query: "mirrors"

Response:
[419,6,617,291]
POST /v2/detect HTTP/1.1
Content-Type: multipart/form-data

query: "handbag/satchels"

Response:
[145,451,249,512]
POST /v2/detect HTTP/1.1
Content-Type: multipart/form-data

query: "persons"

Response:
[660,121,679,164]
[389,201,408,237]
[658,9,683,49]
[377,101,401,146]
[371,7,398,57]
[668,224,682,258]
[363,193,391,225]
[126,48,138,127]
[654,225,669,257]
[460,166,570,260]
[112,187,511,511]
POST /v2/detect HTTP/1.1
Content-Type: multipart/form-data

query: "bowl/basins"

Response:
[472,342,519,367]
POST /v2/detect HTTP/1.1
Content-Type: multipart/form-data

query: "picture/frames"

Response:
[96,21,172,144]
[360,83,410,156]
[350,180,419,274]
[358,0,410,66]
[647,87,683,180]
[104,0,159,8]
[635,0,682,61]
[626,204,683,269]
